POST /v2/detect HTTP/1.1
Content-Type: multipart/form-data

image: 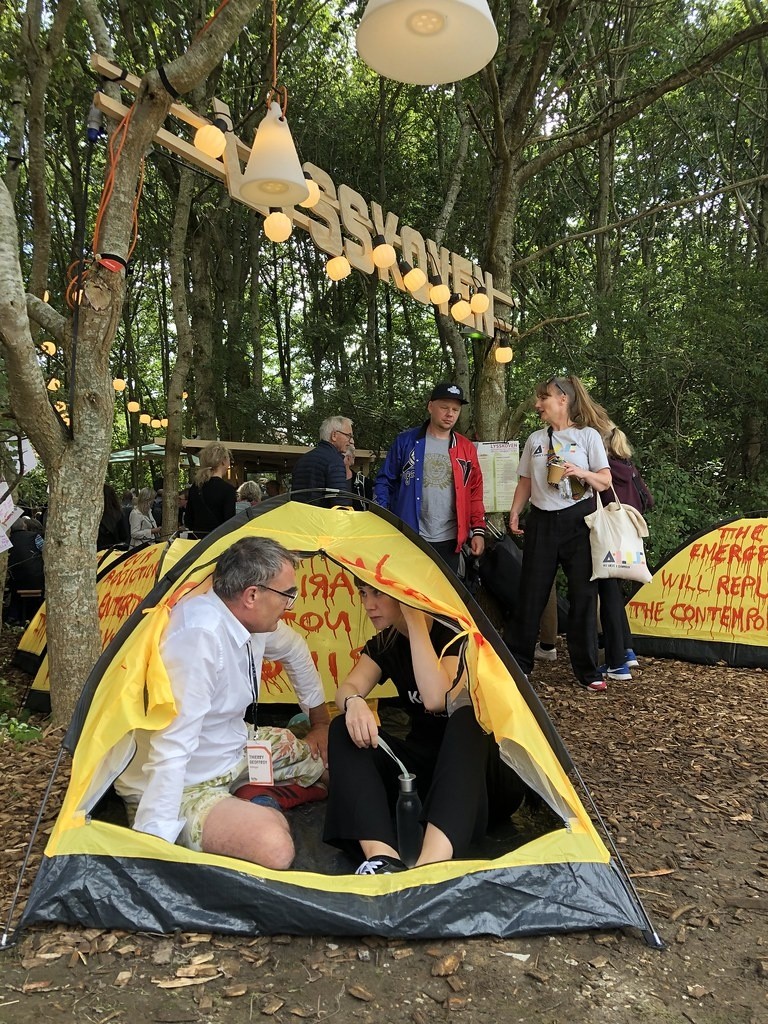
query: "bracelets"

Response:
[344,695,367,711]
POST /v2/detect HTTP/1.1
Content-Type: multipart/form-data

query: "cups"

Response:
[547,462,566,485]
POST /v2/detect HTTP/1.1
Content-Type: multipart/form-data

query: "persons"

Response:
[235,480,261,514]
[7,504,49,623]
[261,480,280,501]
[372,384,486,583]
[323,576,499,875]
[507,375,654,691]
[97,483,191,552]
[183,442,236,540]
[341,444,375,511]
[289,416,354,509]
[114,537,332,870]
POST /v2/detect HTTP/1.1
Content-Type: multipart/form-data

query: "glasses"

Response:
[257,585,299,610]
[545,374,566,395]
[178,498,186,500]
[336,430,354,439]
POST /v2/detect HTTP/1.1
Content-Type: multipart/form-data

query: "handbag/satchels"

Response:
[584,481,654,584]
[589,458,653,515]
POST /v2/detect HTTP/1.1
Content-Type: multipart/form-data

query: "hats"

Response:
[430,383,469,405]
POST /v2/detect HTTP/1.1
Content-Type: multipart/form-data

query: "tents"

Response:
[1,488,667,951]
[17,526,203,717]
[624,510,768,669]
[2,543,137,677]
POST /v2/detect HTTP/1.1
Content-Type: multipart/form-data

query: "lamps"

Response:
[239,102,310,207]
[355,0,499,86]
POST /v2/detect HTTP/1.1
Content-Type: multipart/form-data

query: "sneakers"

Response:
[229,778,330,808]
[577,679,607,691]
[598,665,632,680]
[534,642,557,663]
[624,652,639,669]
[597,648,606,666]
[355,859,409,874]
